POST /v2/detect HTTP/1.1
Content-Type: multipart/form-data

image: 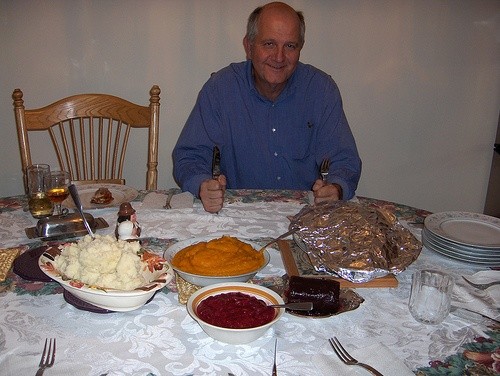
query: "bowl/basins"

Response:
[39,246,174,312]
[163,237,270,286]
[185,282,285,345]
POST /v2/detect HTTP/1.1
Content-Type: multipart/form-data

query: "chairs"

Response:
[13,84,160,194]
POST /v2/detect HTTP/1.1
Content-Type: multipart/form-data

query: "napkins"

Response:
[477,270,500,307]
[312,342,416,376]
[141,191,194,209]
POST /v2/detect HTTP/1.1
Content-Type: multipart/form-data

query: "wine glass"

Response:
[43,171,70,219]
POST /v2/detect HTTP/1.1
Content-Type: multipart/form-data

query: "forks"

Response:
[327,336,383,376]
[320,159,333,188]
[461,276,500,290]
[36,338,56,376]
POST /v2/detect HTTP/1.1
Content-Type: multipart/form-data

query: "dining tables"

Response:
[0,189,500,376]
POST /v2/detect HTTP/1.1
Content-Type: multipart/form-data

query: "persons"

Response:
[173,2,363,213]
[115,202,141,241]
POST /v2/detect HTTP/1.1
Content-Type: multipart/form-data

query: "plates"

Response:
[422,211,500,266]
[61,184,139,210]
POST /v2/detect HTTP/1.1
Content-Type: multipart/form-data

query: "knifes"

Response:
[212,146,221,180]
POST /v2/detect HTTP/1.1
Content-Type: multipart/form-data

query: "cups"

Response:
[26,164,54,219]
[409,269,454,324]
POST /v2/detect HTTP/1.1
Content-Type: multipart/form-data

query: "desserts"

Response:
[282,275,340,312]
